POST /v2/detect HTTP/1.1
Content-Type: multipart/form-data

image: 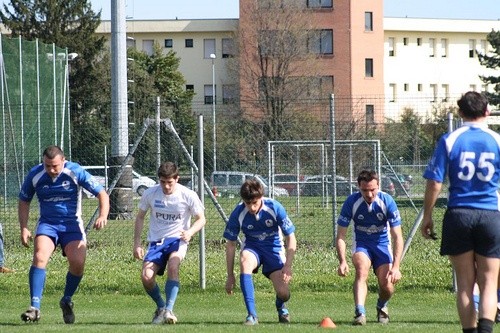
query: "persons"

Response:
[133,162,207,324]
[18,145,109,324]
[335,170,404,325]
[421,91,500,333]
[223,180,296,325]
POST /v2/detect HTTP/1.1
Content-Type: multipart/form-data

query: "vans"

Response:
[79,166,156,196]
[210,171,290,197]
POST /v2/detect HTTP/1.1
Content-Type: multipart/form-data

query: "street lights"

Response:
[209,52,218,171]
[46,52,78,117]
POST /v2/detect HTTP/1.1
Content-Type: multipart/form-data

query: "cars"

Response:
[378,173,413,197]
[265,174,304,195]
[299,175,357,196]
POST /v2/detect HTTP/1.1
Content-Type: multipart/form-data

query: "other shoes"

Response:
[0,266,10,273]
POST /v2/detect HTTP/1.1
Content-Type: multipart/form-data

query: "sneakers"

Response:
[276,301,290,323]
[60,299,75,324]
[152,309,164,324]
[242,317,258,326]
[163,311,178,324]
[377,304,389,323]
[21,308,40,323]
[352,314,366,326]
